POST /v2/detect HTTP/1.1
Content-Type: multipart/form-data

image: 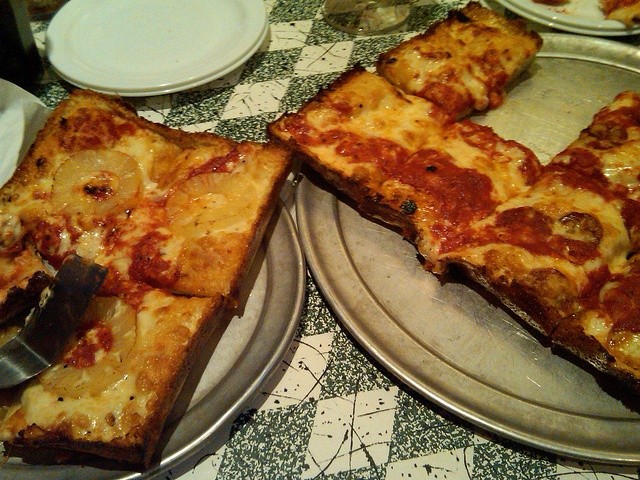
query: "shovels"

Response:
[0,255,109,389]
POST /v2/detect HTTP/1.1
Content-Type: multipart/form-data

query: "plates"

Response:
[126,195,306,472]
[498,0,639,37]
[293,33,640,468]
[47,1,270,98]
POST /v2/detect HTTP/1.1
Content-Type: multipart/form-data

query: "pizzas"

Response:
[1,87,290,468]
[264,0,640,399]
